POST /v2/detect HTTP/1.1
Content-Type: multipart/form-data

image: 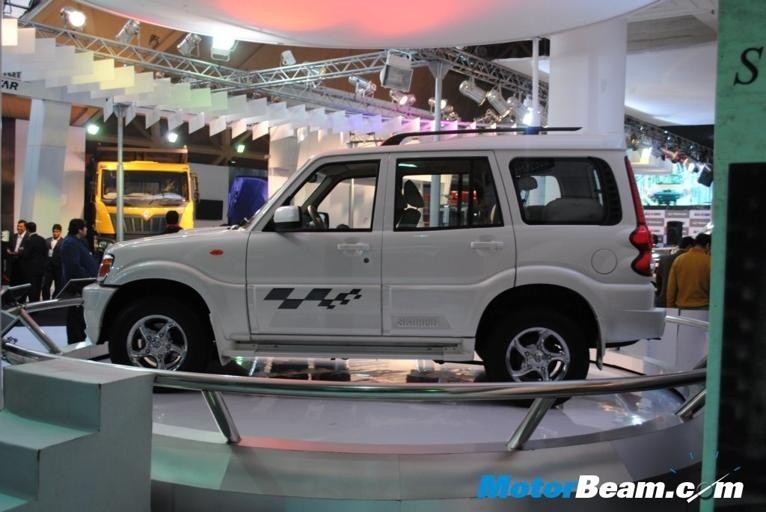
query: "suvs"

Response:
[79,121,671,410]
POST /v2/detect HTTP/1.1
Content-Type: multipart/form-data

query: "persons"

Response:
[1,218,100,303]
[653,238,696,307]
[665,232,711,310]
[160,210,183,235]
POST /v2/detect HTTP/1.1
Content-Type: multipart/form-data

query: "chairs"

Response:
[484,175,603,223]
[399,182,424,227]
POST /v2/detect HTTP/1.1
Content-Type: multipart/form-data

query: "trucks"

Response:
[89,141,200,259]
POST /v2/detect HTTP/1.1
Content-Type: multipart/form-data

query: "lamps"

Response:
[340,53,531,125]
[58,5,201,61]
[279,49,297,67]
